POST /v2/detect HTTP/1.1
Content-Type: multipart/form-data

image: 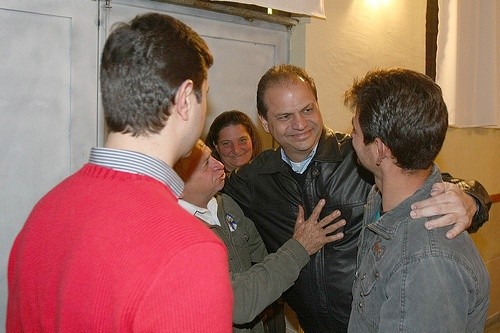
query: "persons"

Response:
[342,66,489,332]
[6,12,234,332]
[205,111,265,179]
[220,63,493,333]
[174,133,346,333]
